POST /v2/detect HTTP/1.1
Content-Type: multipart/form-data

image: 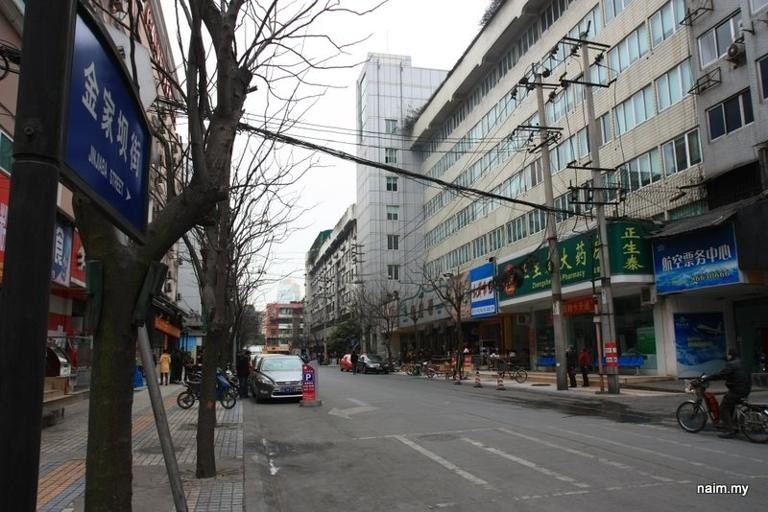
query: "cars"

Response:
[357,353,391,375]
[339,354,353,371]
[248,354,307,403]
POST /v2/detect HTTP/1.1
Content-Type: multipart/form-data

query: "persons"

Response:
[566,346,579,387]
[701,349,750,438]
[579,347,592,386]
[351,351,358,374]
[158,348,194,386]
[236,351,252,398]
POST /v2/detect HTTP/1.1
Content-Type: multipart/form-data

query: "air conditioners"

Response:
[166,271,182,301]
[113,0,128,14]
[161,114,166,120]
[159,155,164,165]
[173,143,178,166]
[726,43,745,58]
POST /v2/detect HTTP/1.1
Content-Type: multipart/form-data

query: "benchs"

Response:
[42,376,72,418]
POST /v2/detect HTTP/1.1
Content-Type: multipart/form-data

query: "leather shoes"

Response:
[718,431,736,438]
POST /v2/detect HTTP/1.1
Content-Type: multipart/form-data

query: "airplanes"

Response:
[697,322,722,335]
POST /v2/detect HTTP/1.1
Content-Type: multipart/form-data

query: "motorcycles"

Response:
[677,373,768,444]
[423,361,455,378]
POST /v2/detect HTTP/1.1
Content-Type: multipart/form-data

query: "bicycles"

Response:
[498,361,528,383]
[178,370,240,410]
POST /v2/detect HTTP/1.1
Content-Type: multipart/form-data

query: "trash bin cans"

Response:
[133,364,143,388]
